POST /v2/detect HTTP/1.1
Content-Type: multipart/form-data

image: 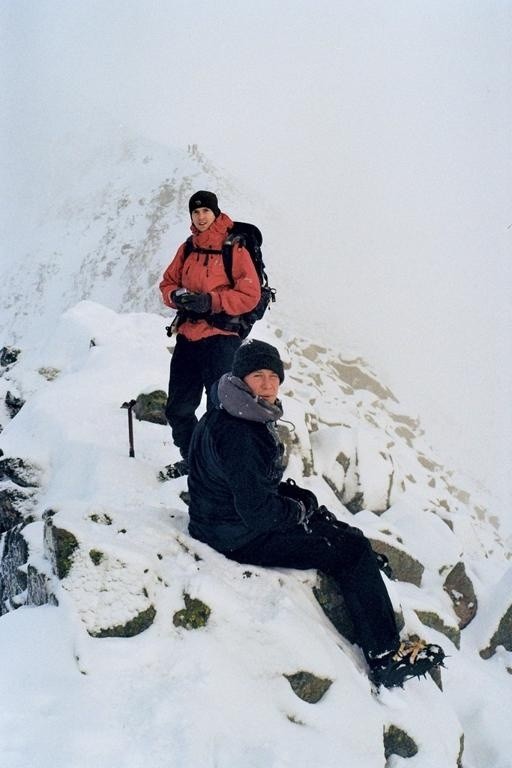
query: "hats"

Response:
[189,191,220,217]
[231,339,284,385]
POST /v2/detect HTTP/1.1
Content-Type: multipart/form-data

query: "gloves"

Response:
[278,478,318,524]
[171,288,211,314]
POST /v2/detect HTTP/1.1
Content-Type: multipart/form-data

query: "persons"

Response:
[158,191,262,481]
[185,340,445,690]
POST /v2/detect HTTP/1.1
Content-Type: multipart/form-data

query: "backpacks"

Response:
[184,222,277,339]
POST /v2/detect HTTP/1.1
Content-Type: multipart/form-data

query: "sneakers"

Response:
[160,459,189,480]
[366,641,445,692]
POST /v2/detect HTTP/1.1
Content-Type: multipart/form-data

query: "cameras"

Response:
[175,287,190,297]
